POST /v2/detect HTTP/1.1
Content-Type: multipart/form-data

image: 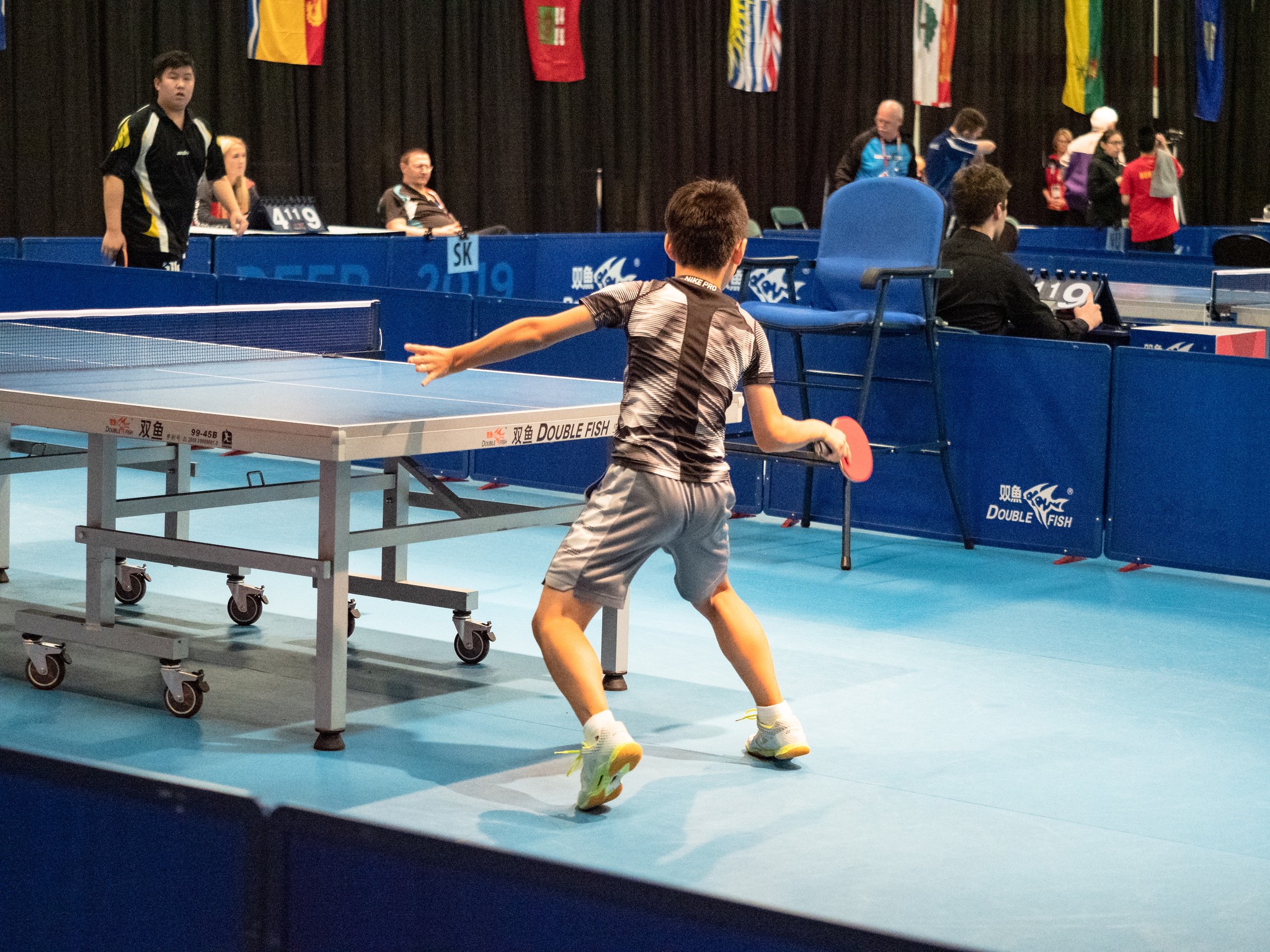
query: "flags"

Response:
[726,0,1227,123]
[247,1,328,66]
[524,0,585,82]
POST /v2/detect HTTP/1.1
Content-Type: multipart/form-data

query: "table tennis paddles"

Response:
[814,416,873,483]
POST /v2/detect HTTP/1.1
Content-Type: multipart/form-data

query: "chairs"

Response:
[722,173,976,568]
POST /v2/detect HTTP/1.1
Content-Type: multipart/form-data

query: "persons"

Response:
[375,148,511,237]
[404,180,850,809]
[922,108,996,239]
[193,135,261,228]
[1119,124,1184,254]
[1039,127,1072,227]
[835,99,917,189]
[932,164,1104,342]
[1059,106,1125,229]
[1086,129,1124,229]
[101,50,250,273]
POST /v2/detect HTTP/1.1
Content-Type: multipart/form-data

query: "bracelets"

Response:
[423,226,433,236]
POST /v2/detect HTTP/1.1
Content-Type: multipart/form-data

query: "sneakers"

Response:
[576,721,643,811]
[746,716,810,761]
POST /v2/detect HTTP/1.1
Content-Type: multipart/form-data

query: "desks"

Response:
[0,320,638,753]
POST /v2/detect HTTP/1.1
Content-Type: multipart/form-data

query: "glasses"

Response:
[1107,140,1124,147]
